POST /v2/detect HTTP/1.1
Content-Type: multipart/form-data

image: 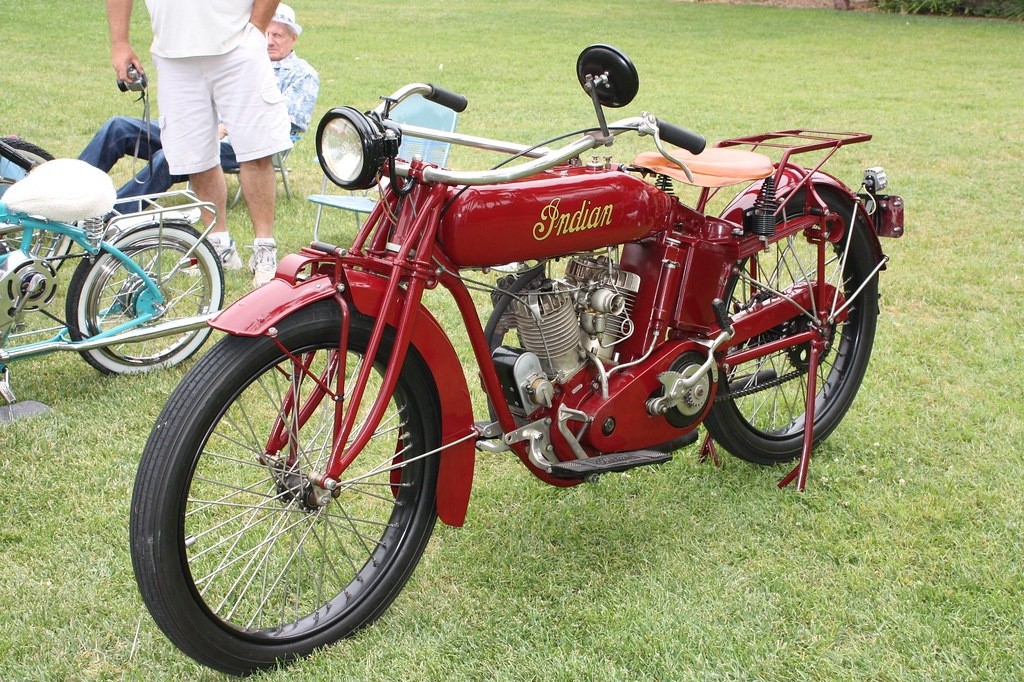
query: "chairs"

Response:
[186,128,302,209]
[308,93,458,242]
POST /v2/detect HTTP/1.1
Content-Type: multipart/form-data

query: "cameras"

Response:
[116,67,147,92]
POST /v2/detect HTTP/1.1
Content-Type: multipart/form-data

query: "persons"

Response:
[77,0,320,287]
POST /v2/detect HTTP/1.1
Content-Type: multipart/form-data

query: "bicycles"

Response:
[1,137,237,405]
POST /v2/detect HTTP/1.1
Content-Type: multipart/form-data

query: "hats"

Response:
[270,2,302,36]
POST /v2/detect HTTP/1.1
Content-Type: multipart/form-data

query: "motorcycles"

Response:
[129,43,906,679]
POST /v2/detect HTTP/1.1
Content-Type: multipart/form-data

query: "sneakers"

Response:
[178,240,243,276]
[248,243,277,289]
